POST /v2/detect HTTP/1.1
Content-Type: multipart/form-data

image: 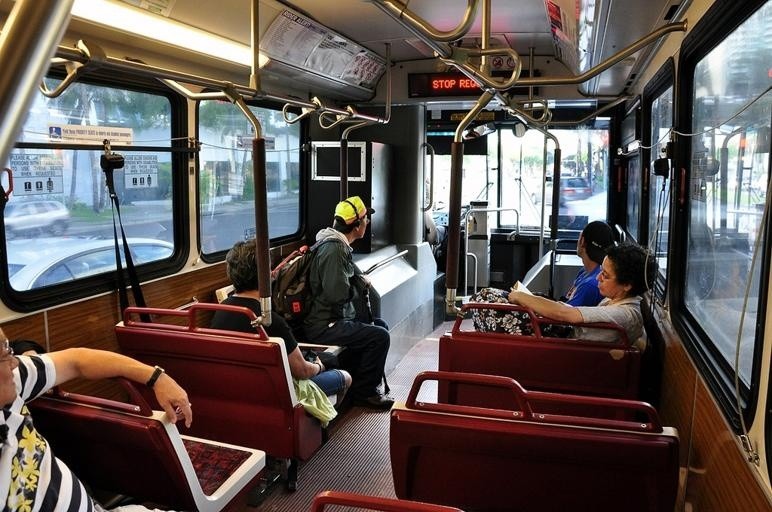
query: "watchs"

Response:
[146,365,166,390]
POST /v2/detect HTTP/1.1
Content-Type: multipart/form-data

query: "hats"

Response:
[583,221,613,265]
[334,196,375,224]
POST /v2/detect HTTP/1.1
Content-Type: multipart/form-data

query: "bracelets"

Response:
[315,363,323,373]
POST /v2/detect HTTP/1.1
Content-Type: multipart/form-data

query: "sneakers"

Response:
[352,390,395,410]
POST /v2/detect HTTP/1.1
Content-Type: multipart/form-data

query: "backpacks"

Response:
[270,238,344,328]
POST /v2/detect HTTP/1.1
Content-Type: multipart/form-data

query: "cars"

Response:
[543,166,571,177]
[2,238,179,288]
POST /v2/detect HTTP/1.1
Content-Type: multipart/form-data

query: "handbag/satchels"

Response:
[302,350,339,368]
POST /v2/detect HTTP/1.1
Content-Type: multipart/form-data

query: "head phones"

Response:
[344,199,361,229]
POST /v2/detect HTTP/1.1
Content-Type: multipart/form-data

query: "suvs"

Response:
[5,200,68,237]
[534,179,592,205]
[561,191,713,289]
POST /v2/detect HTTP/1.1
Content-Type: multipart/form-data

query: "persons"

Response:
[295,195,397,412]
[688,233,764,338]
[0,328,196,512]
[561,220,615,312]
[507,241,659,355]
[423,205,449,245]
[210,239,355,414]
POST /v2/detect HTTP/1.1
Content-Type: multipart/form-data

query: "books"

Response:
[510,280,537,298]
